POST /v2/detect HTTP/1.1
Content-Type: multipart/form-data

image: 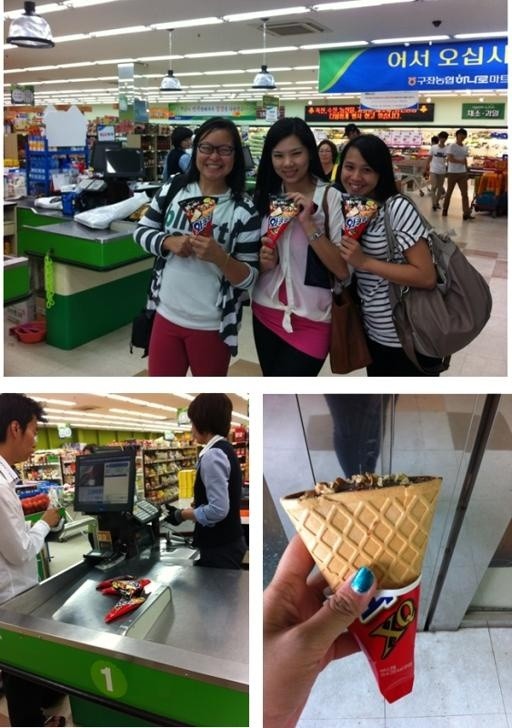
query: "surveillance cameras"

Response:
[432,21,441,27]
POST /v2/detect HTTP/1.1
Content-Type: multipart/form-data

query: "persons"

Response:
[1,393,67,727]
[252,117,351,377]
[342,123,361,139]
[132,115,262,376]
[265,533,376,728]
[162,126,191,181]
[324,395,397,477]
[421,128,475,219]
[340,135,444,377]
[82,446,94,454]
[165,394,246,570]
[317,140,339,181]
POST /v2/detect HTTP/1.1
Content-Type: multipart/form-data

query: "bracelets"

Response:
[223,253,230,265]
[309,231,323,241]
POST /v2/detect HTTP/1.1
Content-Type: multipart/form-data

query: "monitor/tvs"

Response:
[74,452,134,511]
[90,140,122,174]
[104,147,145,177]
[242,145,254,172]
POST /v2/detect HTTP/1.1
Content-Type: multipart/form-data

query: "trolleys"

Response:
[395,159,431,198]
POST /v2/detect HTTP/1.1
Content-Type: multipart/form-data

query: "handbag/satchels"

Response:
[328,288,373,376]
[131,304,157,350]
[303,243,338,289]
[384,193,494,376]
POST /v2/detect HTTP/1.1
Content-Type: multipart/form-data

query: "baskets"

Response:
[14,320,48,343]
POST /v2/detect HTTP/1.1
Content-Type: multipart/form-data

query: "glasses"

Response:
[197,142,233,158]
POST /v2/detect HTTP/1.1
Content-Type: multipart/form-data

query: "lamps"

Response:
[5,1,56,49]
[159,29,183,90]
[251,18,276,88]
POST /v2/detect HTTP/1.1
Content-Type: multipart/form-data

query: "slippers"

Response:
[41,711,68,727]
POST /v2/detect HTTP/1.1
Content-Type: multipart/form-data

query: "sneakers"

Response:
[432,203,476,221]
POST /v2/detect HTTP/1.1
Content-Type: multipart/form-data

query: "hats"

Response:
[343,124,358,135]
